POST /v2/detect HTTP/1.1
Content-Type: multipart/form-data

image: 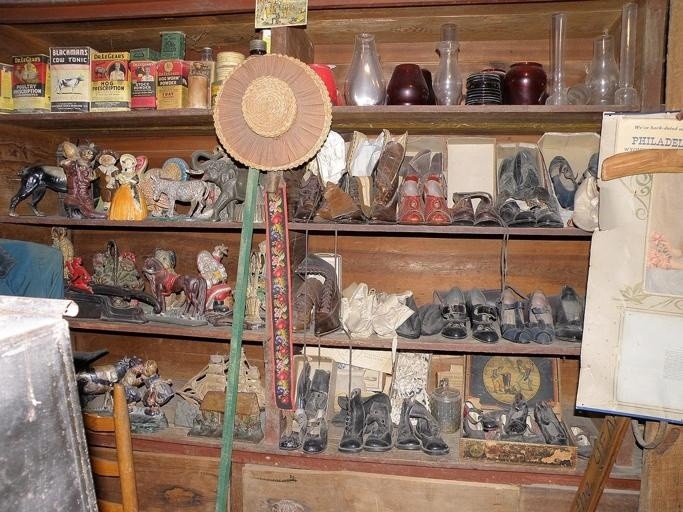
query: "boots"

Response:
[61,163,108,219]
[290,252,341,339]
[278,361,451,456]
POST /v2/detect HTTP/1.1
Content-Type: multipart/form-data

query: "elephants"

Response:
[190,145,249,222]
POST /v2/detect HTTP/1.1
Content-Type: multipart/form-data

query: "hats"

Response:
[211,53,335,174]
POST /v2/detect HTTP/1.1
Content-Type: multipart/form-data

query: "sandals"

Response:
[567,424,596,462]
[463,390,570,447]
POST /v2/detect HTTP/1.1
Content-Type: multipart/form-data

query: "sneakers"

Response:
[549,149,598,210]
[341,280,449,344]
[284,130,565,229]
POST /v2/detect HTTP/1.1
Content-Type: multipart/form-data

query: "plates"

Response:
[464,68,506,107]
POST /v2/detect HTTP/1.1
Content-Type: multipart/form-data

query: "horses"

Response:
[142,256,207,320]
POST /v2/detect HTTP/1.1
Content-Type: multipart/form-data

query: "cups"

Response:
[303,62,337,105]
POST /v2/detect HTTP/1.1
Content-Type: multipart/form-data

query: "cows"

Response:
[57,73,85,94]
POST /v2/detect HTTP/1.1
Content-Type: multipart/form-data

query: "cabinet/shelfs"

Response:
[1,0,682,511]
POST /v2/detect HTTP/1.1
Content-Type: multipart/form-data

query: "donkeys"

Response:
[150,169,209,217]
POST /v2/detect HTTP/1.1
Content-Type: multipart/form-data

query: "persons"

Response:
[141,67,154,81]
[109,62,124,81]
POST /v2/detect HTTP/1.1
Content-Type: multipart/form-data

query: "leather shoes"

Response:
[440,285,585,344]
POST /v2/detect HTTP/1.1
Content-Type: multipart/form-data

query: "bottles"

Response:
[248,39,265,55]
[433,24,463,105]
[546,3,637,104]
[196,46,214,108]
[503,63,547,106]
[344,32,428,108]
